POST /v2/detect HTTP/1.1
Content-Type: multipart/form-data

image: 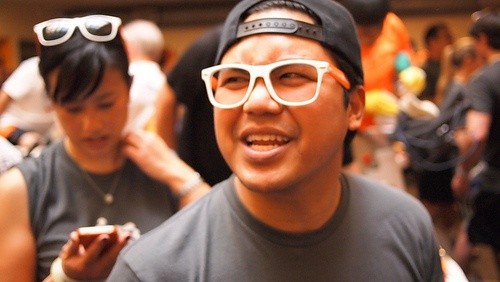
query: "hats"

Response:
[212,0,362,86]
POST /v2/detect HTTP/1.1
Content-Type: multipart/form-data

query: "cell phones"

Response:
[78,226,119,255]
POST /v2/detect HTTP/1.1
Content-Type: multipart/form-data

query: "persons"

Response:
[106,0,444,282]
[121,19,169,136]
[1,15,212,282]
[0,56,66,177]
[342,5,500,282]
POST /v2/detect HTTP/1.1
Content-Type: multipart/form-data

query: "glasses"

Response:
[33,14,124,46]
[200,55,354,108]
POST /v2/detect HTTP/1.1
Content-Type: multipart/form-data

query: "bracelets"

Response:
[51,257,78,282]
[173,172,203,197]
[6,127,25,145]
